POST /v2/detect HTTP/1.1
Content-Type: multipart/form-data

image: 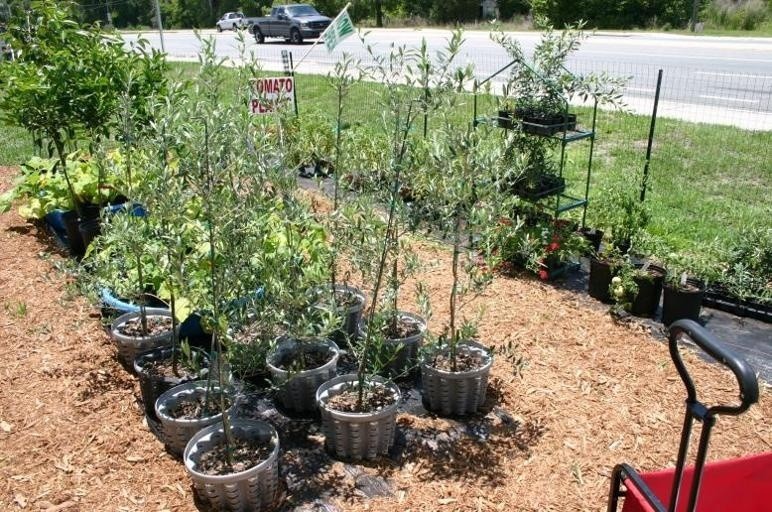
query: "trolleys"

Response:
[601,318,771,512]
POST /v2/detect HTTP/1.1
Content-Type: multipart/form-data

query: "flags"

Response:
[322,12,357,55]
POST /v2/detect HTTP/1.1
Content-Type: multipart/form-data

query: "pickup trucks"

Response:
[215,12,249,34]
[247,3,333,45]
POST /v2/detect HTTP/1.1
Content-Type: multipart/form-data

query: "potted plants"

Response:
[0,0,710,511]
